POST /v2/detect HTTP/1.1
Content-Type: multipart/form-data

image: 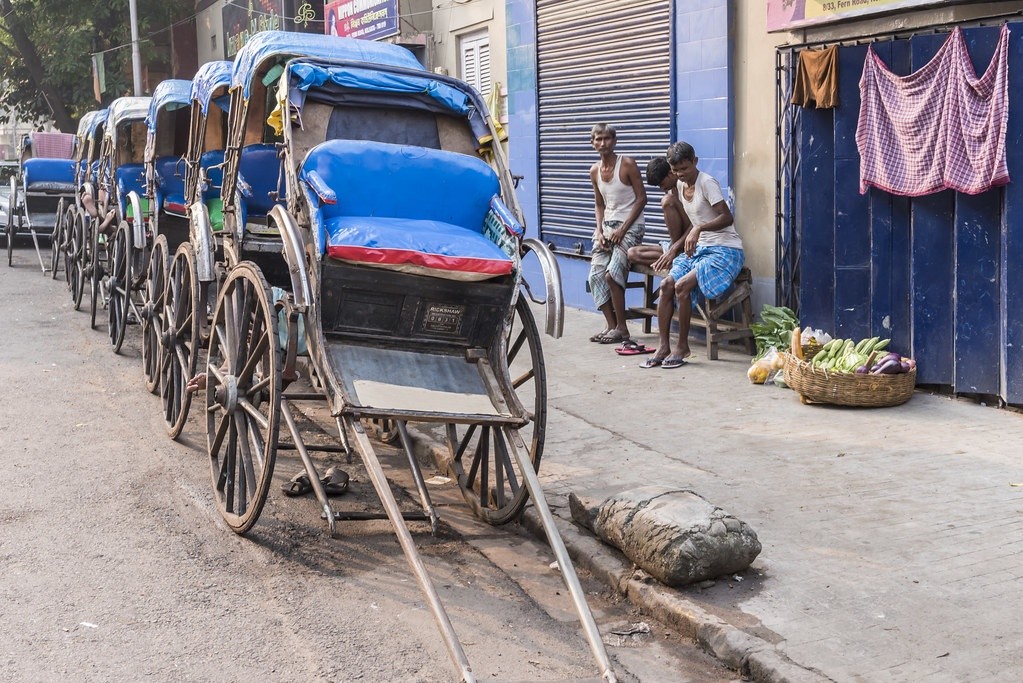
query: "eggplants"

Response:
[855,353,911,373]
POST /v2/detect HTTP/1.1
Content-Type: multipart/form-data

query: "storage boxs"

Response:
[784,345,916,406]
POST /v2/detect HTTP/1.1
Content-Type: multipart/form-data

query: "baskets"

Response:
[785,344,917,407]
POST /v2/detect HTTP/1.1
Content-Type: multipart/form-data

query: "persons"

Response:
[638,141,744,369]
[584,123,647,343]
[627,157,691,270]
[327,8,338,37]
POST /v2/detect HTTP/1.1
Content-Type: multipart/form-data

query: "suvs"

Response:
[0,159,55,240]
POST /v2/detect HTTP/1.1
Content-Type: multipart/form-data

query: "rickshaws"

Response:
[6,33,617,682]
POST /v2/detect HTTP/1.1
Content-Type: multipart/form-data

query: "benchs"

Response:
[154,156,187,217]
[116,164,149,222]
[298,139,525,281]
[22,158,76,193]
[585,263,758,359]
[199,149,224,232]
[232,143,286,241]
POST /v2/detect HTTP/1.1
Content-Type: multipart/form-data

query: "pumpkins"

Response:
[748,362,770,383]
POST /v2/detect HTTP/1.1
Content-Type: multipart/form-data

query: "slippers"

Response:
[280,469,318,498]
[589,328,609,342]
[640,357,664,368]
[321,467,349,493]
[661,357,688,368]
[615,340,656,354]
[601,329,629,343]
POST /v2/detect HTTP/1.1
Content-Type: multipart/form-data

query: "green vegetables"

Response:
[748,304,800,364]
